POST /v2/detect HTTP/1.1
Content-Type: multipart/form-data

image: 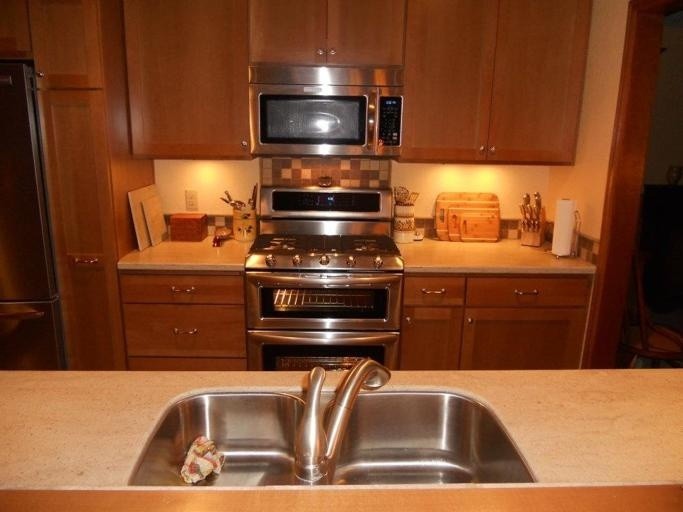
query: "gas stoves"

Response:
[243,234,404,273]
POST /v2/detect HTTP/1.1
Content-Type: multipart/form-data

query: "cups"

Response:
[393,204,415,245]
[231,210,257,241]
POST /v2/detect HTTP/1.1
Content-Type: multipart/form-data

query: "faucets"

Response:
[294,357,391,485]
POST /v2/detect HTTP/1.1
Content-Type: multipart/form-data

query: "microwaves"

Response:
[247,84,404,157]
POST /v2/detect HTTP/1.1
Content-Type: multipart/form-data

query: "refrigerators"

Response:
[0,64,64,371]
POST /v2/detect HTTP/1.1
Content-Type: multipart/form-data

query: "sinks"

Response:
[324,391,536,484]
[128,391,324,487]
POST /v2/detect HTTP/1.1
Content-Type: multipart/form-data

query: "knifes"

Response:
[518,191,542,231]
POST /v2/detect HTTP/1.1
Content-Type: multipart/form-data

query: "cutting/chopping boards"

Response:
[434,190,500,242]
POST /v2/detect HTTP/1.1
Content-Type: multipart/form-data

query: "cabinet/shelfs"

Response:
[27,0,125,89]
[119,273,247,371]
[251,1,408,64]
[461,277,592,371]
[126,1,251,158]
[400,276,465,370]
[395,0,595,164]
[29,89,155,371]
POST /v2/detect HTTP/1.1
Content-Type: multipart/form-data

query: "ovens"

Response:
[243,271,402,373]
